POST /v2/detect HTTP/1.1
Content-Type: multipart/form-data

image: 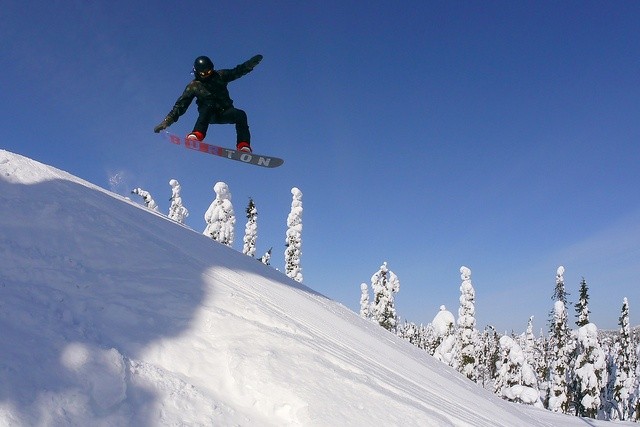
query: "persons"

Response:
[153,55,262,153]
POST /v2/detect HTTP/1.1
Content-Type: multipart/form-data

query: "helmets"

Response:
[194,56,214,81]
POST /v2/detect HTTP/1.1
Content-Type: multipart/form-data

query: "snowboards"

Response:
[159,130,284,168]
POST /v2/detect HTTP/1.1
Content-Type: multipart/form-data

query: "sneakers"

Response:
[240,146,250,153]
[187,134,198,140]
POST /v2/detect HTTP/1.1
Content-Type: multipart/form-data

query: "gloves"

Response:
[154,115,174,133]
[244,54,263,70]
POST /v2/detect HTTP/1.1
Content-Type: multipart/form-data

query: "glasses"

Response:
[199,71,211,76]
